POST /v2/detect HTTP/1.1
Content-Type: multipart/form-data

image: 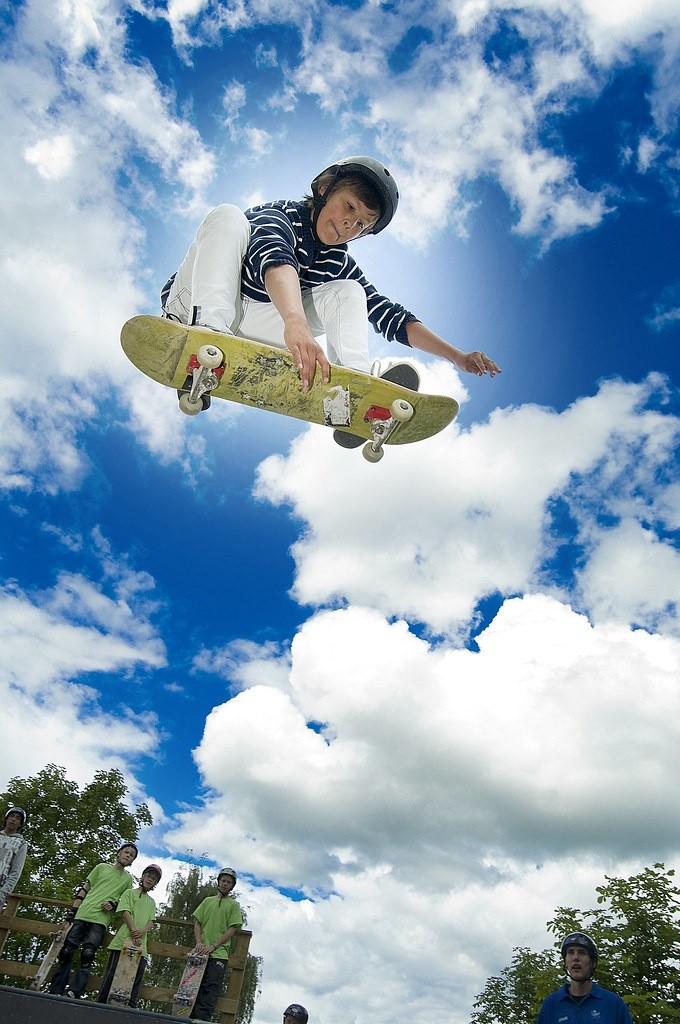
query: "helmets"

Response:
[311,156,399,235]
[147,864,162,880]
[117,842,138,858]
[4,807,26,826]
[219,868,238,879]
[561,933,599,963]
[283,1004,308,1024]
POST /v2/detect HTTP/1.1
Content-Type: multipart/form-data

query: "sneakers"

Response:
[334,360,421,448]
[177,390,211,411]
[64,990,79,998]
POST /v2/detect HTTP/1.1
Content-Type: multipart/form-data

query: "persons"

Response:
[45,841,137,1001]
[282,1003,309,1024]
[97,863,163,1008]
[538,931,632,1024]
[191,866,244,1021]
[159,156,501,451]
[0,807,28,910]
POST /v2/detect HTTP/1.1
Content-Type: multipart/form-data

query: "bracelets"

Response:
[210,943,217,948]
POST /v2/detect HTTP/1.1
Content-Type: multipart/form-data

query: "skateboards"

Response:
[119,313,460,463]
